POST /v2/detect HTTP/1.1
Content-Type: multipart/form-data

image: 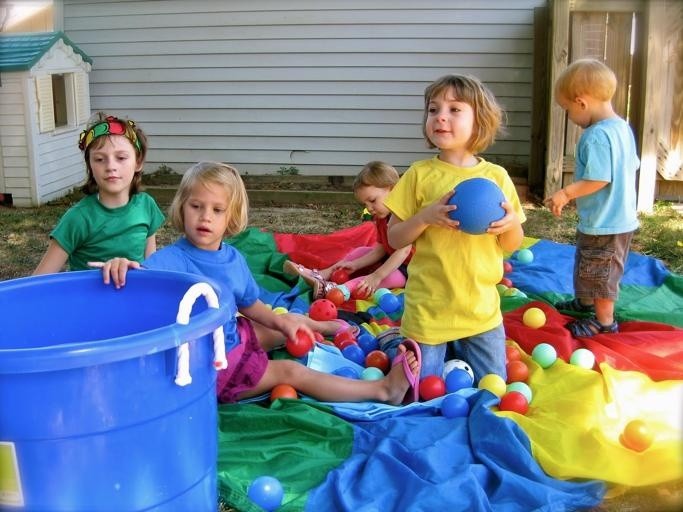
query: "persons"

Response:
[84,158,422,408]
[540,58,642,338]
[382,73,530,385]
[32,111,169,278]
[283,161,416,301]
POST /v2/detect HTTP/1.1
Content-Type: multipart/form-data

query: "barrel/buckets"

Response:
[3,272,237,510]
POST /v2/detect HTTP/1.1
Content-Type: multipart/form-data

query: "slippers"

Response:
[392,338,422,401]
[323,319,360,337]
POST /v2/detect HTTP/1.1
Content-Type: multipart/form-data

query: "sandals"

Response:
[283,259,337,301]
[554,298,619,336]
